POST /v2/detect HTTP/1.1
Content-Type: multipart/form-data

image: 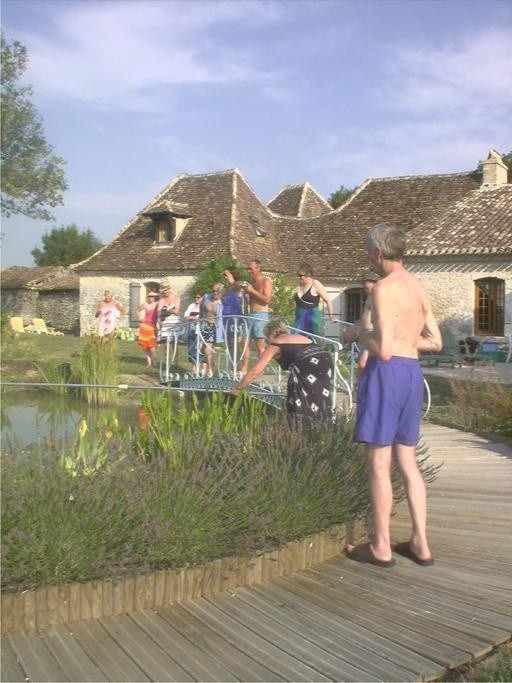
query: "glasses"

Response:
[298,274,306,277]
[194,297,200,299]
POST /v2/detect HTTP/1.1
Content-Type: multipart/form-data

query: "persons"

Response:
[133,260,271,378]
[344,224,444,565]
[230,319,334,423]
[292,264,338,343]
[94,290,126,359]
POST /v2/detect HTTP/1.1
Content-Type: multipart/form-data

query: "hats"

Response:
[159,281,171,292]
[147,291,158,297]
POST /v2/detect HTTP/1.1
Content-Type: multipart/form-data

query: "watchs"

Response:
[235,385,242,392]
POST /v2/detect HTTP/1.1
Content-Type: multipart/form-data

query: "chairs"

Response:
[421,322,470,368]
[8,315,64,335]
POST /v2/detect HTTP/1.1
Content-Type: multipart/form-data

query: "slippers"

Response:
[348,544,397,568]
[392,542,436,567]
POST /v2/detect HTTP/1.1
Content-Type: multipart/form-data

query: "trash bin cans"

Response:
[458,337,508,362]
